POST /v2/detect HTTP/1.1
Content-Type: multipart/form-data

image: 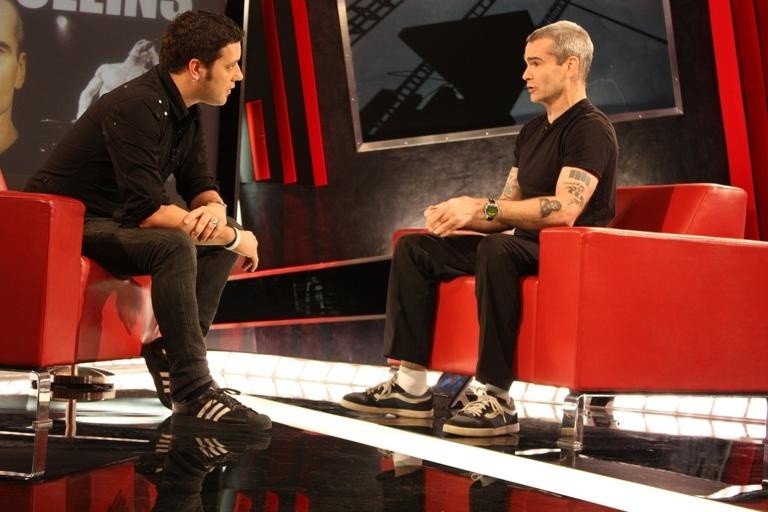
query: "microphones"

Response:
[139,40,154,53]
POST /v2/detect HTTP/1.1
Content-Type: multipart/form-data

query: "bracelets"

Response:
[225,226,239,252]
[203,198,227,208]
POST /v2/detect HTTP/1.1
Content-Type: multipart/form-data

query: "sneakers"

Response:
[173,387,272,431]
[142,336,172,409]
[339,371,434,418]
[442,387,521,438]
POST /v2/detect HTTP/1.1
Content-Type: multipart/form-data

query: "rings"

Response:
[210,219,219,228]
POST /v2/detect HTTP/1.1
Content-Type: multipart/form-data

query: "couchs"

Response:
[390,181,767,467]
[0,167,167,480]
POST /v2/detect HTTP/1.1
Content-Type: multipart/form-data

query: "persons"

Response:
[340,20,620,438]
[340,405,520,511]
[2,2,53,183]
[141,431,275,511]
[78,39,160,117]
[24,9,274,431]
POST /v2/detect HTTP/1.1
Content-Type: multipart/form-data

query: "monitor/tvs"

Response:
[0,0,249,223]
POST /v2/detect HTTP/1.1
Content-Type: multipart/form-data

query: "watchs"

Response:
[485,196,498,221]
